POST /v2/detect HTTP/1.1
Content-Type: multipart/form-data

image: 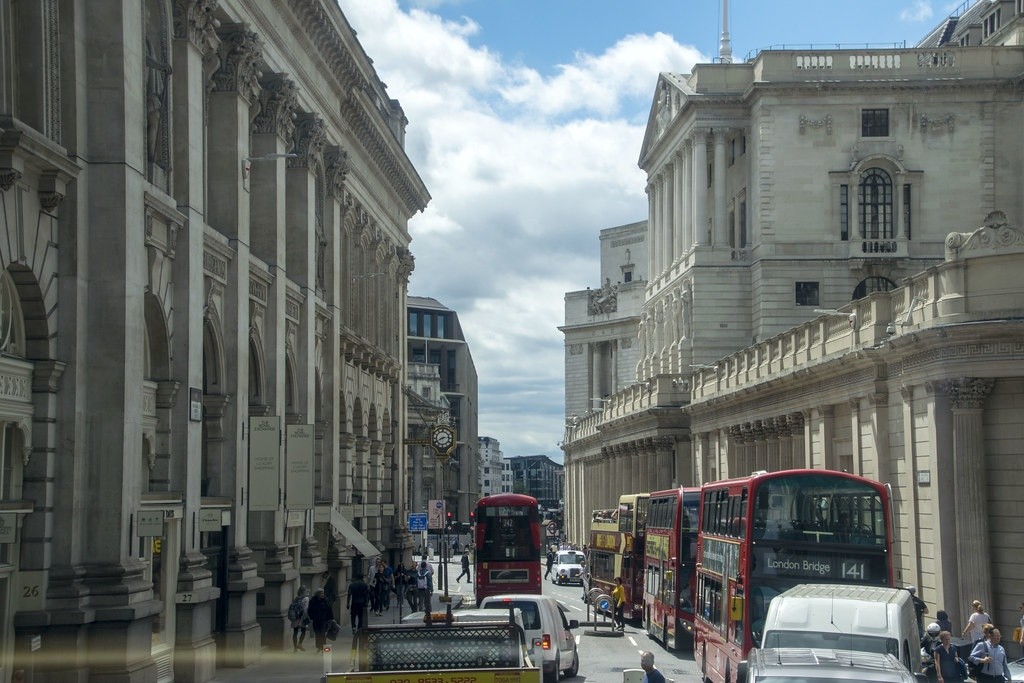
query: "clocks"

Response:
[431,426,457,455]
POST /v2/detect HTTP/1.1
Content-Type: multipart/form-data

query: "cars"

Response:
[479,593,580,683]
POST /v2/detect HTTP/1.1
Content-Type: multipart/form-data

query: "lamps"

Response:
[689,364,718,372]
[562,425,576,428]
[590,398,611,405]
[813,310,857,322]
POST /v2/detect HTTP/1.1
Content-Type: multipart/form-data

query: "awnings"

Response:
[317,510,382,560]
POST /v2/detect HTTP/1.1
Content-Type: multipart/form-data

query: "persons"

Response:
[347,575,370,634]
[641,652,665,683]
[612,577,626,631]
[394,561,433,611]
[576,561,586,599]
[422,548,429,560]
[962,601,1011,683]
[428,542,433,547]
[456,550,472,584]
[369,558,395,614]
[920,610,958,683]
[907,587,929,637]
[1019,603,1024,627]
[836,513,869,534]
[545,549,553,580]
[453,537,459,555]
[323,573,336,605]
[292,587,309,652]
[550,543,588,556]
[307,589,333,653]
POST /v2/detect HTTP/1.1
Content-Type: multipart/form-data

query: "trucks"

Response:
[323,607,541,683]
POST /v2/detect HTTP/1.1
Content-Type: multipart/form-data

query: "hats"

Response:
[906,586,915,592]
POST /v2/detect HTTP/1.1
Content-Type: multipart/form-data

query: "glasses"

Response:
[1019,607,1024,609]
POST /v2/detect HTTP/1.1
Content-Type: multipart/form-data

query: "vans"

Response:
[551,549,587,587]
[737,583,935,683]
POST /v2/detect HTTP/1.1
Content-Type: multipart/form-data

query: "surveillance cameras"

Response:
[886,326,896,336]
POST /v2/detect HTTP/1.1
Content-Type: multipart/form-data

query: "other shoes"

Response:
[297,646,305,651]
[456,579,460,583]
[467,580,472,583]
[615,626,624,631]
[352,628,356,634]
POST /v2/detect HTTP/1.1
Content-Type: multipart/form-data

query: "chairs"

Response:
[718,519,875,544]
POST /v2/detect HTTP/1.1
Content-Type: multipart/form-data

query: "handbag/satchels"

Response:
[968,638,988,676]
[326,623,341,640]
[303,618,310,626]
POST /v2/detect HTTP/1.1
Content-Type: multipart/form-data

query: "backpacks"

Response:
[289,596,305,621]
[417,570,429,589]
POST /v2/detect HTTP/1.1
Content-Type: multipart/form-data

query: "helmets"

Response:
[927,623,941,642]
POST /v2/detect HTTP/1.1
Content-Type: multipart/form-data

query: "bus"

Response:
[587,493,650,624]
[471,493,544,607]
[693,468,896,683]
[642,484,705,653]
